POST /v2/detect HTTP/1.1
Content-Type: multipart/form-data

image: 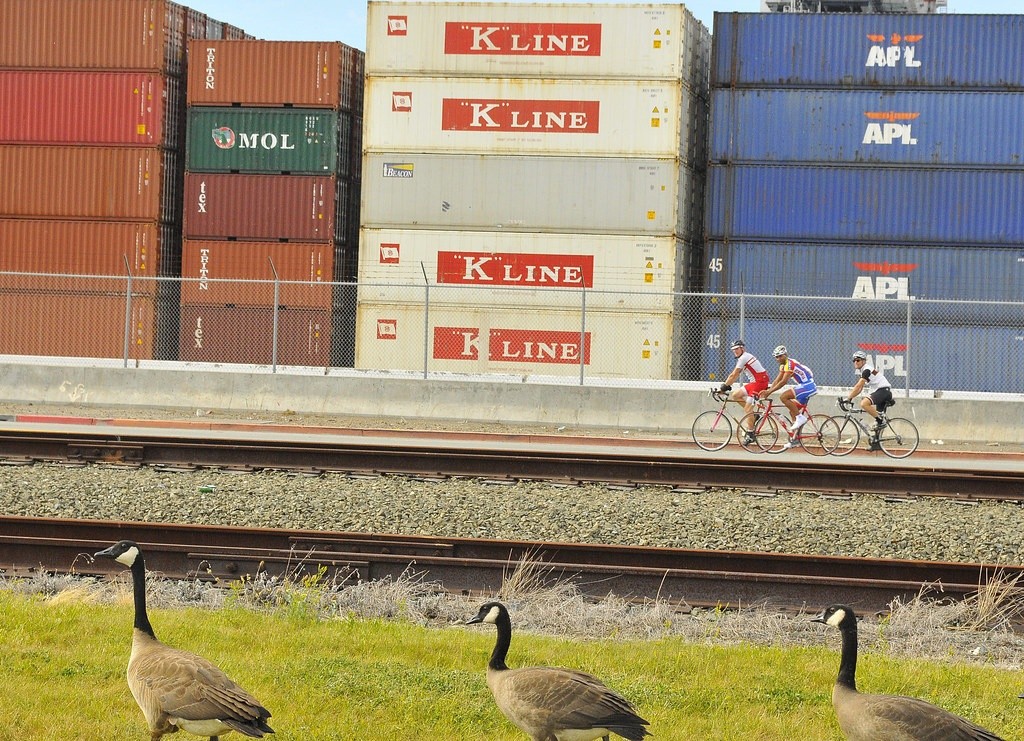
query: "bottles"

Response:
[860,419,871,432]
[778,413,793,428]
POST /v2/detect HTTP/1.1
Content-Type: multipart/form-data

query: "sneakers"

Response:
[871,421,887,431]
[791,415,808,430]
[865,444,881,450]
[742,437,756,444]
[754,414,760,425]
[784,439,802,447]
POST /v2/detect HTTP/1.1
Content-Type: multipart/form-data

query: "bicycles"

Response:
[736,387,841,457]
[816,397,920,459]
[690,387,779,455]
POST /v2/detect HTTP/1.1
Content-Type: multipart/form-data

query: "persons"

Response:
[715,340,769,446]
[844,351,892,451]
[758,346,818,447]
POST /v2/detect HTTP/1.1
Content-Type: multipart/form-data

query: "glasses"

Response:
[853,359,863,362]
[776,354,784,359]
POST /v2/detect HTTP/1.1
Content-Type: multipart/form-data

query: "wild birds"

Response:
[93,539,275,741]
[811,605,1004,741]
[465,602,654,741]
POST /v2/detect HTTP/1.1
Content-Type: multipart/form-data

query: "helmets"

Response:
[730,339,745,350]
[772,346,787,357]
[853,351,867,359]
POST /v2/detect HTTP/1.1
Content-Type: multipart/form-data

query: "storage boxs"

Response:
[0,0,1024,396]
